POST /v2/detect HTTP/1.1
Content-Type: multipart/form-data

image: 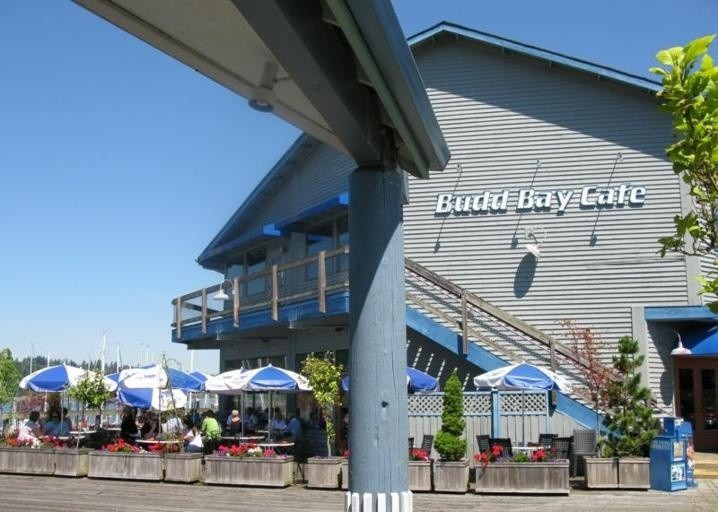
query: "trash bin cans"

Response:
[649,416,699,492]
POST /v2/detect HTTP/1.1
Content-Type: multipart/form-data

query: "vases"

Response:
[88,451,164,482]
[341,461,430,491]
[473,462,570,495]
[204,455,293,488]
[1,446,65,475]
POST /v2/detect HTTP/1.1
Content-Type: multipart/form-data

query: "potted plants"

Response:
[162,353,203,483]
[297,350,348,489]
[53,368,111,479]
[563,320,619,492]
[600,333,660,492]
[430,365,474,495]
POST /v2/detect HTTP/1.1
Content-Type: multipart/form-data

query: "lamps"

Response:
[212,277,236,301]
[660,325,693,357]
[520,221,550,258]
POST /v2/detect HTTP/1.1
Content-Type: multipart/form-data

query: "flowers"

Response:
[409,447,430,462]
[5,429,66,450]
[210,440,287,460]
[474,441,570,463]
[100,436,163,454]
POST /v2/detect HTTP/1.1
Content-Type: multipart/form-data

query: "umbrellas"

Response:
[688,322,718,355]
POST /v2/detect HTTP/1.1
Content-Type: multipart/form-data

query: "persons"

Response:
[274,429,294,455]
[180,415,205,453]
[19,410,48,446]
